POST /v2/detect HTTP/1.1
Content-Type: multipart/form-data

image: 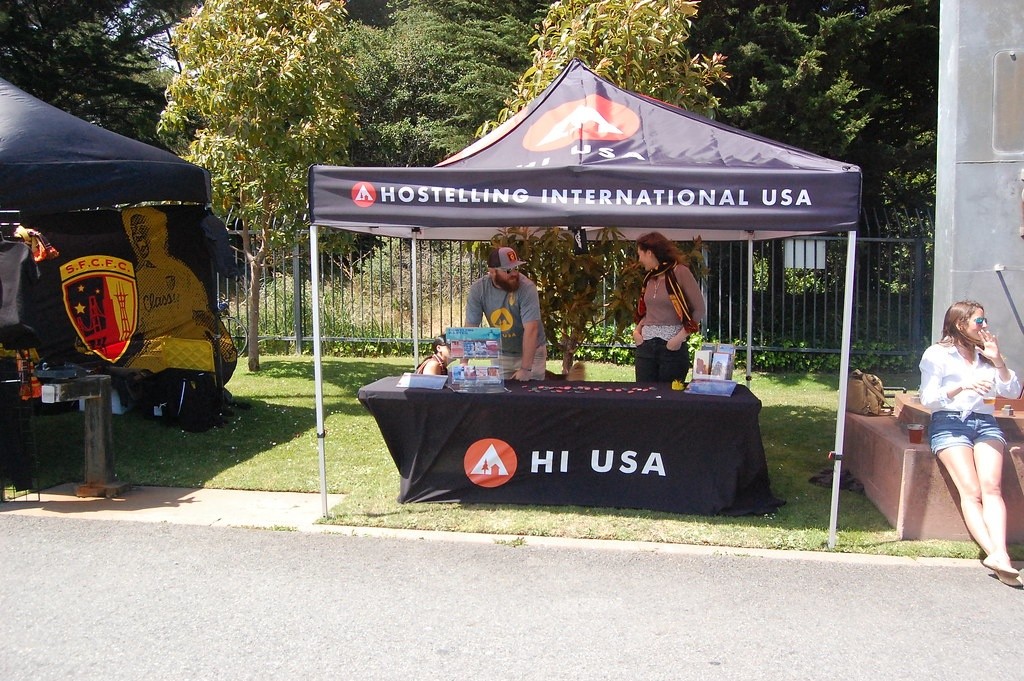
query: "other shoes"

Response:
[984,557,1019,578]
[995,570,1022,587]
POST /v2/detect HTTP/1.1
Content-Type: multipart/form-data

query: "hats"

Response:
[488,246,526,268]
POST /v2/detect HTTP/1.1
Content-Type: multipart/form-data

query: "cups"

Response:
[907,424,924,443]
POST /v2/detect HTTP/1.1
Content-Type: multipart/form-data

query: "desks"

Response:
[357,375,787,518]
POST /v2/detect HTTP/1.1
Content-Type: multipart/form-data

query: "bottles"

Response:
[0,343,41,401]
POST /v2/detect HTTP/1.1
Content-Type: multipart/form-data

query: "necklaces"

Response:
[653,276,663,299]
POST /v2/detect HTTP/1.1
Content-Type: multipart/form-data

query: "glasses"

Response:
[498,266,518,274]
[967,317,987,325]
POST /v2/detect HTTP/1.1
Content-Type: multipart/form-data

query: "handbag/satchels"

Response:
[846,369,893,416]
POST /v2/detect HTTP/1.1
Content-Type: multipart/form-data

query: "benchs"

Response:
[839,392,1024,545]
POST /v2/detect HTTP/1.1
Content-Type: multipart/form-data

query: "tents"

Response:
[306,58,864,549]
[0,77,228,427]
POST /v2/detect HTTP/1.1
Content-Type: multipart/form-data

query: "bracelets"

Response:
[521,366,533,372]
[996,362,1005,368]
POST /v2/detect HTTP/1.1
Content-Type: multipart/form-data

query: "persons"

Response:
[919,300,1023,587]
[463,247,547,380]
[413,334,457,374]
[632,231,706,383]
